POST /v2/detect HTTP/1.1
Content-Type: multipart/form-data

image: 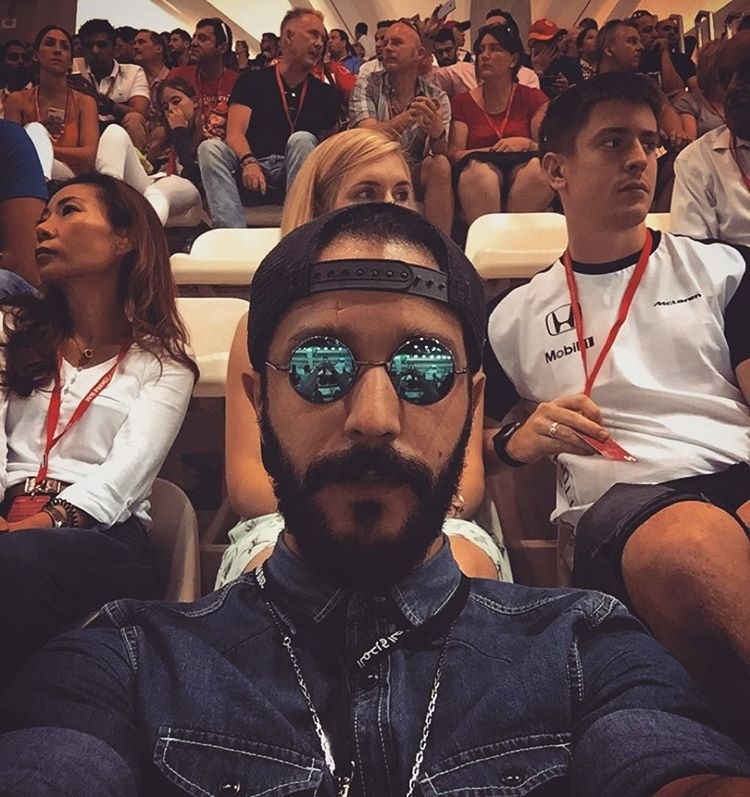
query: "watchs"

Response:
[492,422,527,467]
[44,506,70,528]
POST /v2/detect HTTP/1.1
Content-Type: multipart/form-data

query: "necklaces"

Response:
[72,336,96,357]
[265,601,455,797]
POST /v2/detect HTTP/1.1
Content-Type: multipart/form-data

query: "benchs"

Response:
[163,209,672,591]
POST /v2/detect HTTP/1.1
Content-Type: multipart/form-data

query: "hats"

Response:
[247,202,486,365]
[446,19,470,30]
[527,18,567,47]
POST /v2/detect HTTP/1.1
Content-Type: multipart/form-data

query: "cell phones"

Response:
[540,76,563,86]
[66,72,85,87]
[47,106,65,122]
[438,0,456,20]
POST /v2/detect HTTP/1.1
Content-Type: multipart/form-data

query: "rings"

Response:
[550,422,558,437]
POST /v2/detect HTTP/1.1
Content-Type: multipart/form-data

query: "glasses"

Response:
[211,17,229,44]
[263,337,473,406]
[632,11,648,19]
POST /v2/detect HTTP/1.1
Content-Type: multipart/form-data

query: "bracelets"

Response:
[424,33,433,39]
[243,160,257,167]
[418,375,422,379]
[316,366,321,371]
[400,372,404,376]
[48,498,78,528]
[432,129,446,141]
[332,370,336,373]
[241,153,256,162]
[453,497,463,515]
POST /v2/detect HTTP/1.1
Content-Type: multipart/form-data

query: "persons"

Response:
[291,361,349,401]
[0,202,750,796]
[392,366,451,402]
[0,4,750,300]
[216,127,507,588]
[485,72,750,688]
[0,173,198,694]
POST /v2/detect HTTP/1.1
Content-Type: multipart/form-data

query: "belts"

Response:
[5,478,66,496]
